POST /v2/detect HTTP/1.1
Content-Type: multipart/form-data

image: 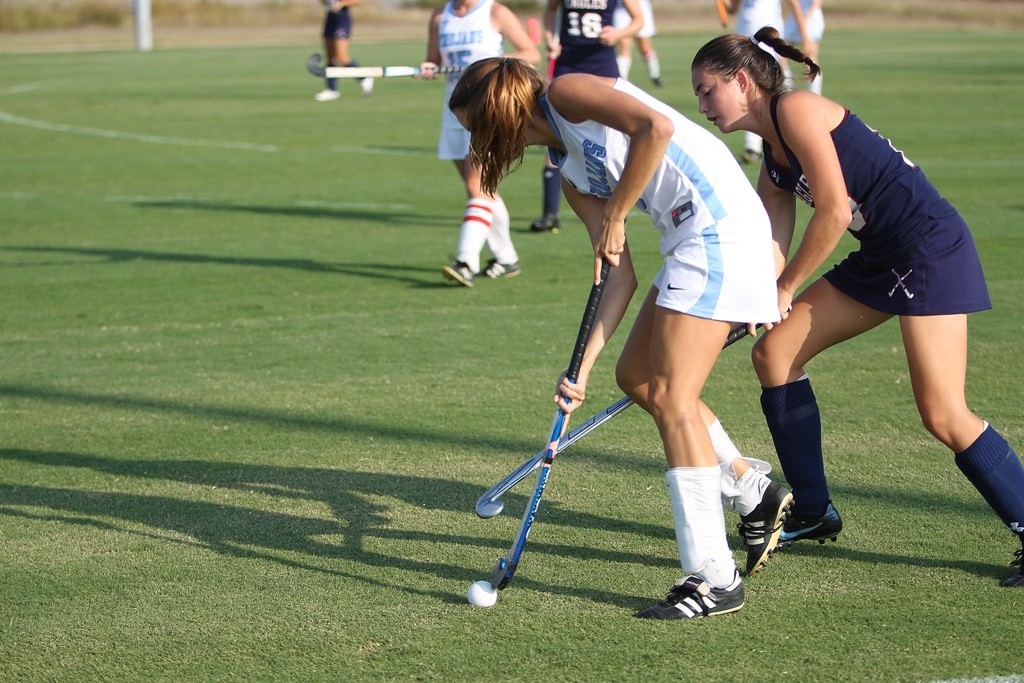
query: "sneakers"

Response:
[441,260,473,287]
[999,547,1024,588]
[776,499,842,549]
[737,476,795,576]
[475,259,521,281]
[631,568,745,620]
[530,217,558,232]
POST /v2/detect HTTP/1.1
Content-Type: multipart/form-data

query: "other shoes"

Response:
[314,89,341,101]
[360,77,374,94]
[652,77,662,86]
[742,148,762,164]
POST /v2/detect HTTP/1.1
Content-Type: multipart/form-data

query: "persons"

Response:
[529,0,665,232]
[411,0,543,287]
[690,26,1024,587]
[448,56,794,620]
[722,0,825,164]
[314,0,375,101]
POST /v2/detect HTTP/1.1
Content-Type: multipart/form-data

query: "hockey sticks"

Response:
[492,255,611,590]
[307,53,468,79]
[475,304,792,519]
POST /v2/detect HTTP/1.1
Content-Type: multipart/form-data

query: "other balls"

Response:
[467,581,498,607]
